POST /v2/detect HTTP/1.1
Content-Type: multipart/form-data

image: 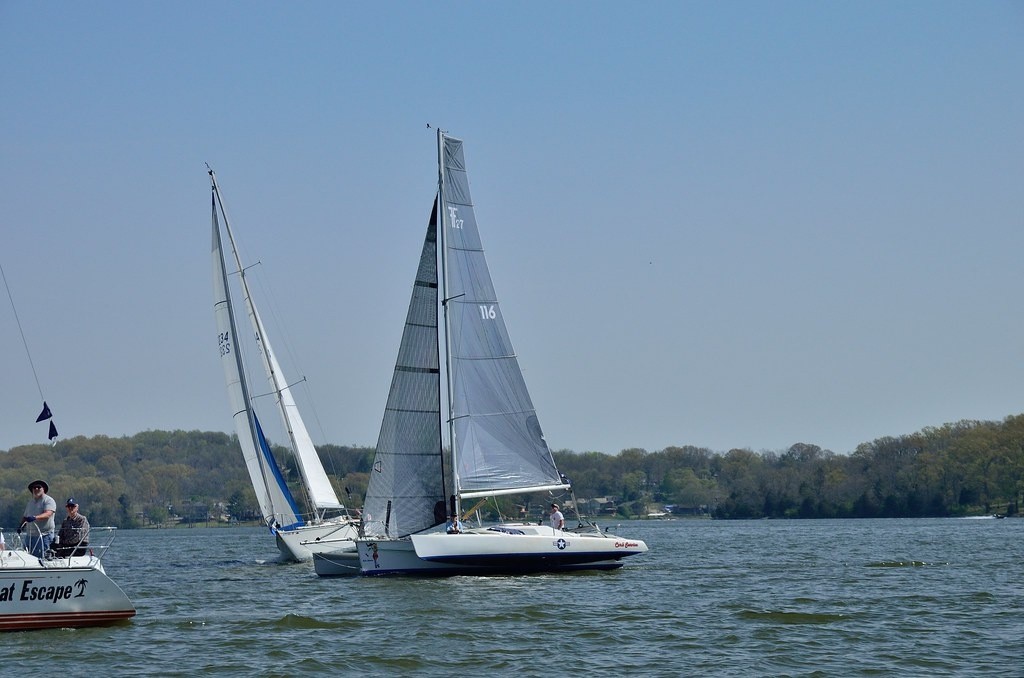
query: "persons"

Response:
[550,504,564,531]
[17,480,56,558]
[446,512,463,534]
[0,532,5,551]
[50,497,90,557]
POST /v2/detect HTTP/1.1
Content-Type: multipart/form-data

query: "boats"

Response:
[0,523,137,632]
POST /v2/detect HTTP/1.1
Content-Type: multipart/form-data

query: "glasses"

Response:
[33,485,43,489]
[66,504,76,508]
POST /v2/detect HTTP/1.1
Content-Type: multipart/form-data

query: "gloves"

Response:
[17,526,23,534]
[27,516,36,522]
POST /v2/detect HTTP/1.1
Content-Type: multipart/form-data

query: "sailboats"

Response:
[313,128,650,577]
[203,158,359,561]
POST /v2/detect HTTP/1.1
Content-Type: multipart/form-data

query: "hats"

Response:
[551,503,559,509]
[28,480,48,493]
[65,497,76,506]
[450,512,458,515]
